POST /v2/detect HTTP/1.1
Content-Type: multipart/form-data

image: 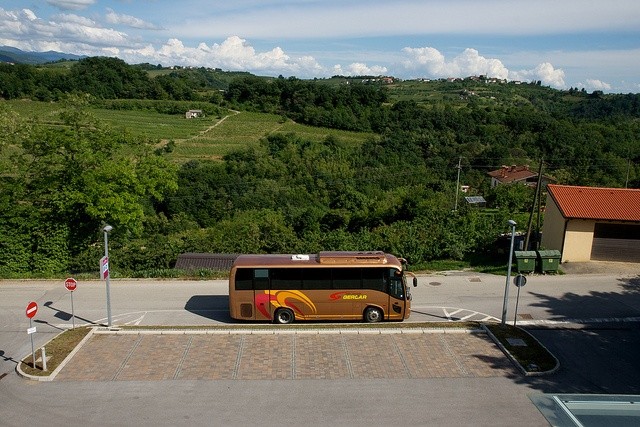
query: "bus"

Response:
[229,251,418,323]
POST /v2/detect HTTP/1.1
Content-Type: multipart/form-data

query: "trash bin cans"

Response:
[537,250,561,275]
[515,251,537,275]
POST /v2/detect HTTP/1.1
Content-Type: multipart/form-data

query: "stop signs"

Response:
[64,278,78,291]
[26,301,38,319]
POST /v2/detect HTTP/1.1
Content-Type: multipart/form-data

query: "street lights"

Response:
[501,220,518,326]
[103,225,113,326]
